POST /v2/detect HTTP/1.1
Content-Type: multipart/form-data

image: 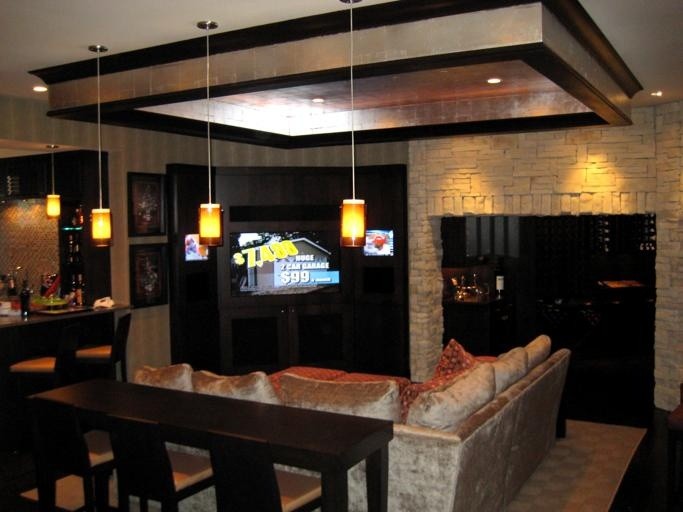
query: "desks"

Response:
[25,379,393,512]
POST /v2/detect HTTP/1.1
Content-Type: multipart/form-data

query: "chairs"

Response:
[93,414,218,512]
[8,322,88,457]
[25,398,128,512]
[75,313,131,383]
[208,433,321,512]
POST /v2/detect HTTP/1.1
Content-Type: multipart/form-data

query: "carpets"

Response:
[503,418,648,512]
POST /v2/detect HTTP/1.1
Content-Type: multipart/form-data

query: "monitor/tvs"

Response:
[228,230,340,298]
[363,229,395,257]
[184,233,209,262]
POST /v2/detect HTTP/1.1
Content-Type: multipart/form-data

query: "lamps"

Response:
[88,44,113,247]
[196,21,224,246]
[46,145,61,219]
[341,1,366,248]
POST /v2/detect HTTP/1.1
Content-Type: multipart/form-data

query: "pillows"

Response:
[133,334,551,433]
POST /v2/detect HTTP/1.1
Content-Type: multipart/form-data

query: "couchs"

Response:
[347,334,570,511]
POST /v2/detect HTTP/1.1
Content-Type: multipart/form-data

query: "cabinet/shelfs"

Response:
[167,162,409,379]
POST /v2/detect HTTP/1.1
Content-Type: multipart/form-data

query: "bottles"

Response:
[66,203,85,264]
[0,266,88,324]
[6,173,19,197]
[451,272,481,302]
[494,267,505,302]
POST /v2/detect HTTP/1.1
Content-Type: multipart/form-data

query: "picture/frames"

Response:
[129,242,170,310]
[127,170,167,237]
